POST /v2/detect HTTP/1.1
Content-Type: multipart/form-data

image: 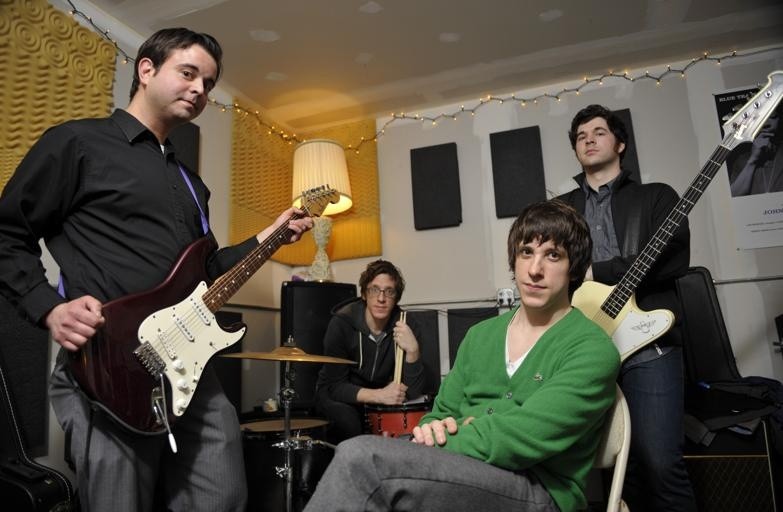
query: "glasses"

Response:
[365,286,398,298]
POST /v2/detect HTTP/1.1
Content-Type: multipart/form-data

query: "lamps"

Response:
[291,141,353,282]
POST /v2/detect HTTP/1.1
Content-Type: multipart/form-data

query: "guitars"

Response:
[68,183,340,455]
[571,70,779,363]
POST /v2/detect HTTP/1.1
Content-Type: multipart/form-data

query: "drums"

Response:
[239,417,332,512]
[365,402,430,438]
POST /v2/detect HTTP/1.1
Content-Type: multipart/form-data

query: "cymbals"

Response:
[219,348,358,365]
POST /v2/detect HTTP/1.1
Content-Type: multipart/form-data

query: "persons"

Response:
[555,104,696,510]
[315,260,428,434]
[301,200,621,512]
[729,105,783,196]
[2,27,314,511]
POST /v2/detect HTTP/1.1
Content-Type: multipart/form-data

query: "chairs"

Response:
[0,290,81,512]
[264,278,358,484]
[675,264,783,511]
